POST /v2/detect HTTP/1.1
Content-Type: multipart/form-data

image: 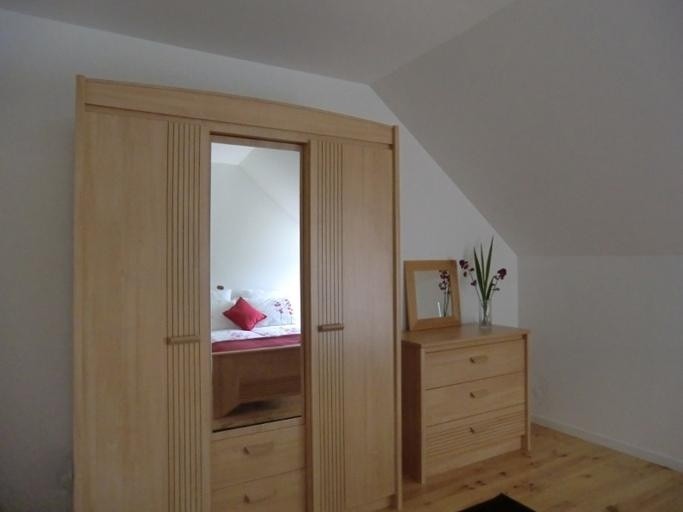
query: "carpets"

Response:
[457,491,537,512]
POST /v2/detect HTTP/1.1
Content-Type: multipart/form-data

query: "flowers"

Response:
[459,233,507,325]
[435,267,449,317]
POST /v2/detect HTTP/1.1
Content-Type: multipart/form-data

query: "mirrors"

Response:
[401,258,463,333]
[205,138,307,433]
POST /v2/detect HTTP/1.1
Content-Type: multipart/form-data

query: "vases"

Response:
[436,300,449,317]
[477,297,492,328]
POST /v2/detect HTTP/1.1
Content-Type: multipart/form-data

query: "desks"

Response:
[401,320,532,488]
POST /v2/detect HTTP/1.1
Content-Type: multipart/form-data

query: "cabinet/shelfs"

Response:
[69,73,405,511]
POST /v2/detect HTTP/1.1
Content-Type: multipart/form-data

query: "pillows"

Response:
[209,288,296,331]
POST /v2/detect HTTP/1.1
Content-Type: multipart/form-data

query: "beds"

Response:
[210,324,303,419]
